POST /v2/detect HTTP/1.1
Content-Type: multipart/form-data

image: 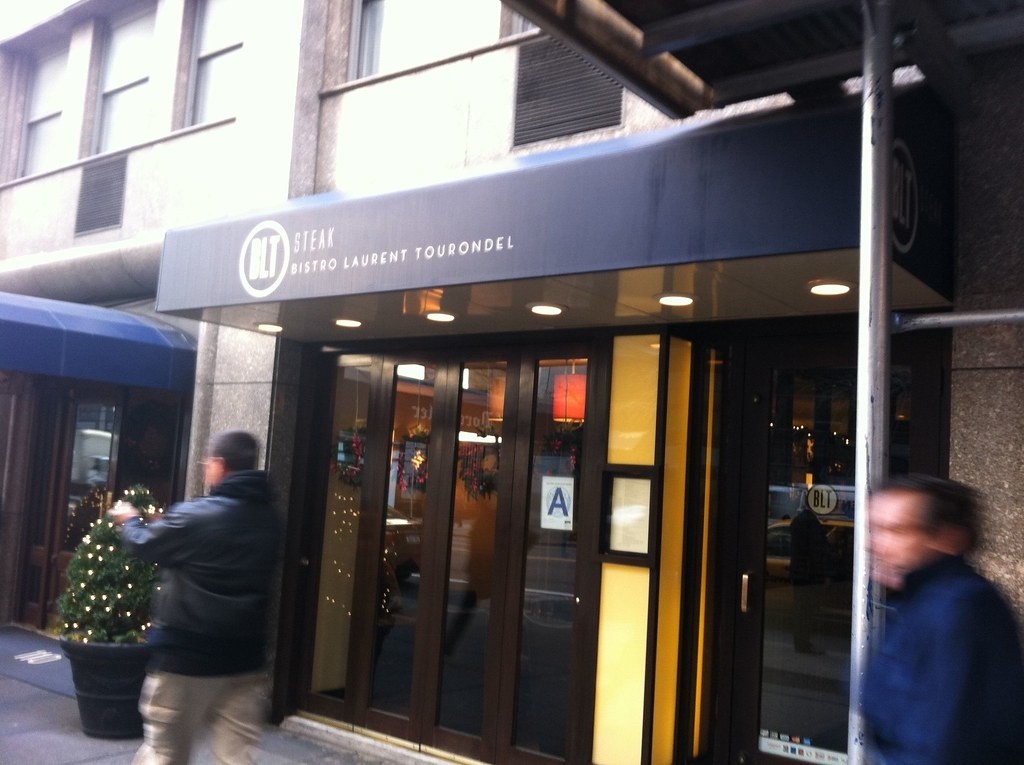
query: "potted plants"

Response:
[57,484,165,741]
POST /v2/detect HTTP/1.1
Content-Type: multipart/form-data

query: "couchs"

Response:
[377,555,479,670]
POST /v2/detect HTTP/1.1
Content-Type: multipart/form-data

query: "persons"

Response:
[859,475,1024,765]
[371,561,402,666]
[789,493,832,655]
[446,490,536,659]
[107,431,280,765]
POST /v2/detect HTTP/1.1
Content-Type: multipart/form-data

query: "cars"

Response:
[765,520,855,632]
